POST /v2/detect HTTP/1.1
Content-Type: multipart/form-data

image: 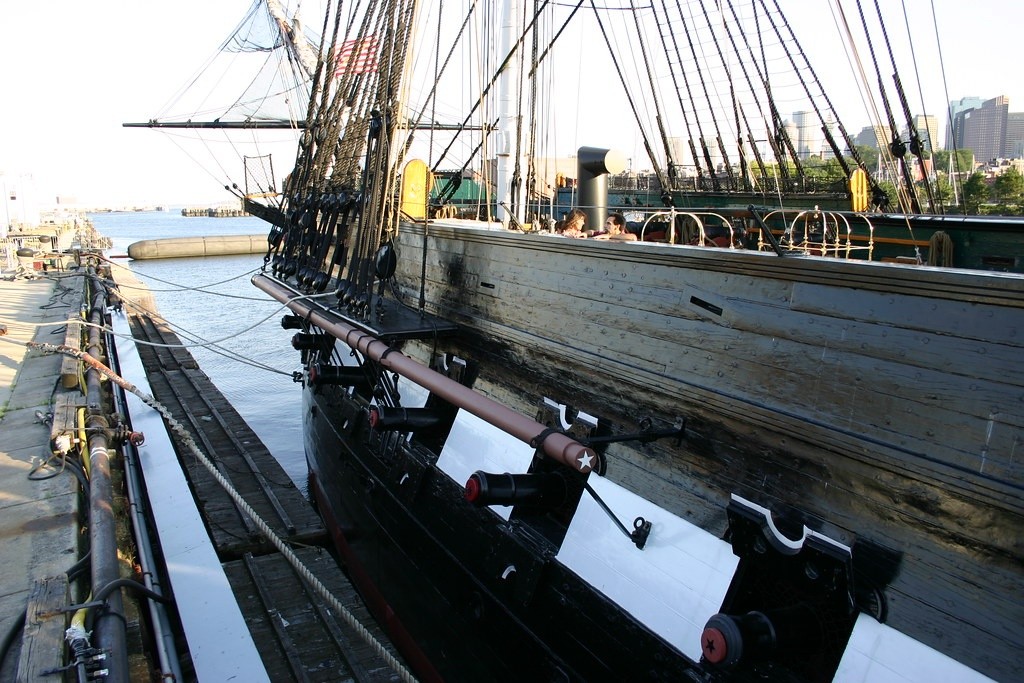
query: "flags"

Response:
[334,35,378,76]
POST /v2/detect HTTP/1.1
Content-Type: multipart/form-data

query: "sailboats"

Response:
[123,0,1024,682]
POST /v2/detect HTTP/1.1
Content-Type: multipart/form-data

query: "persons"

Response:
[576,215,637,241]
[555,209,587,238]
